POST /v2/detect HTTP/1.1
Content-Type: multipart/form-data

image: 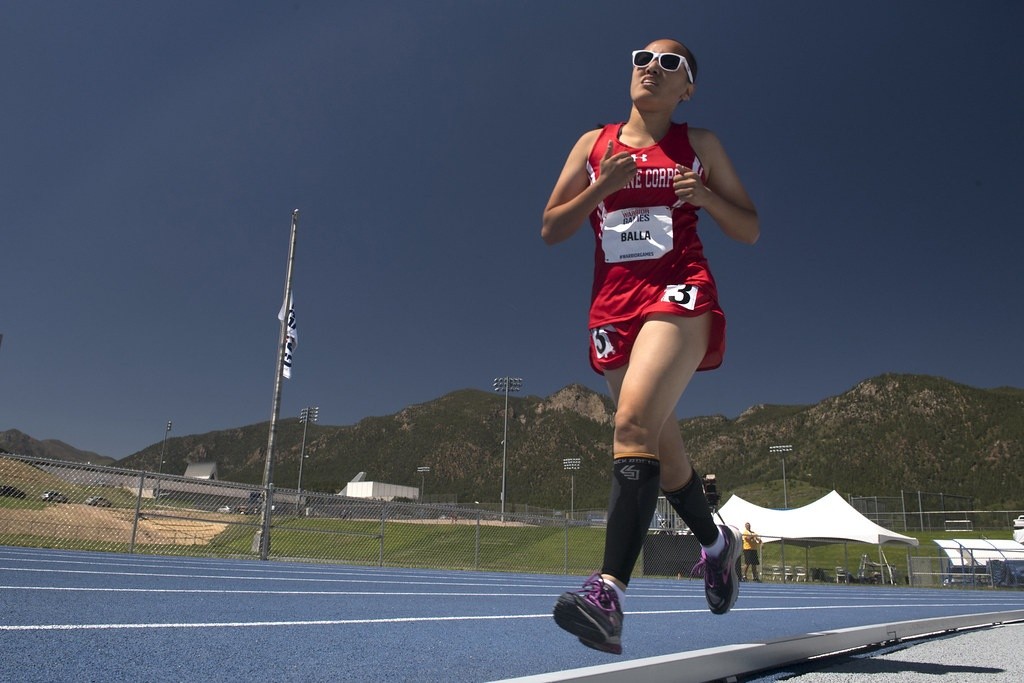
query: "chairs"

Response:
[771,565,850,584]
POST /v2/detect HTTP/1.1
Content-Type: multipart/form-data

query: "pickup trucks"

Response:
[1013,515,1024,529]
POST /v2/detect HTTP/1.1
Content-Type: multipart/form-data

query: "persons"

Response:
[742,523,762,583]
[540,37,761,654]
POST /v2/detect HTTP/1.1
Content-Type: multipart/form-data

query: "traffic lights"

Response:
[704,477,720,508]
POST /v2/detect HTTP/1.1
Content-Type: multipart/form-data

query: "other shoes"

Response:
[742,576,749,582]
[753,578,761,583]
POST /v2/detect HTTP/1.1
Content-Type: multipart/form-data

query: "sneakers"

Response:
[689,524,743,614]
[553,573,623,655]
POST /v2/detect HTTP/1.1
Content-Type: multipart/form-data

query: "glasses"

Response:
[632,50,693,84]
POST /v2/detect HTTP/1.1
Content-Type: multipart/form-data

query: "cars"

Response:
[40,490,68,503]
[238,505,248,514]
[84,496,111,508]
[0,485,26,499]
[217,506,233,515]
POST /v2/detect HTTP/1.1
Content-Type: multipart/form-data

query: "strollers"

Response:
[654,509,673,535]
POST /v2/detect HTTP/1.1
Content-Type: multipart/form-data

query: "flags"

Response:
[277,289,299,379]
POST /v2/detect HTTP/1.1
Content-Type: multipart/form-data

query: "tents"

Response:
[710,490,919,584]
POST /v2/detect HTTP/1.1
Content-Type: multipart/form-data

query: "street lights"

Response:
[770,444,793,508]
[417,465,431,505]
[294,406,320,513]
[562,458,581,519]
[156,420,172,499]
[490,375,526,524]
